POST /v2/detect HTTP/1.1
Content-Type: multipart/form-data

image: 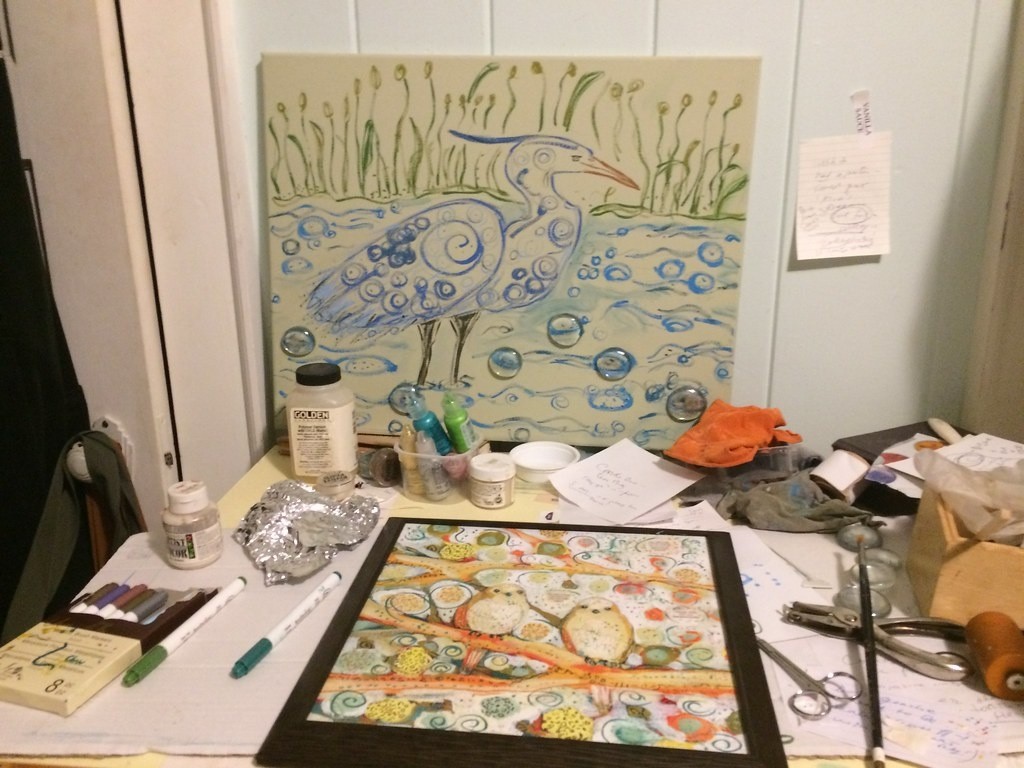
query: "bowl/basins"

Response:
[714,444,800,487]
[511,440,581,483]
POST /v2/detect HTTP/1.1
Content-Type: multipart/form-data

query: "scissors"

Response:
[757,635,864,721]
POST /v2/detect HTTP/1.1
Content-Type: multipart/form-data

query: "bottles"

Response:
[287,363,358,485]
[396,391,477,502]
[160,481,223,570]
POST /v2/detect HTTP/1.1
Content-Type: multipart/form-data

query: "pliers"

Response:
[787,601,973,682]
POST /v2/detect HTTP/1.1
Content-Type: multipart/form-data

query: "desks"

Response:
[0,442,1024,768]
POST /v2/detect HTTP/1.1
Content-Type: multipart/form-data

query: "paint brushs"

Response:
[856,535,886,768]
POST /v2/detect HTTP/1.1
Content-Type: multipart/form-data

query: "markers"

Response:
[121,576,247,688]
[69,582,168,625]
[231,572,342,678]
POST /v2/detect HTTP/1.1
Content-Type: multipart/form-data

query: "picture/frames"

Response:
[256,514,788,768]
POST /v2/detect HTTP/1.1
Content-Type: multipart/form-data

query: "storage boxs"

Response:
[906,489,1024,622]
[0,588,223,719]
